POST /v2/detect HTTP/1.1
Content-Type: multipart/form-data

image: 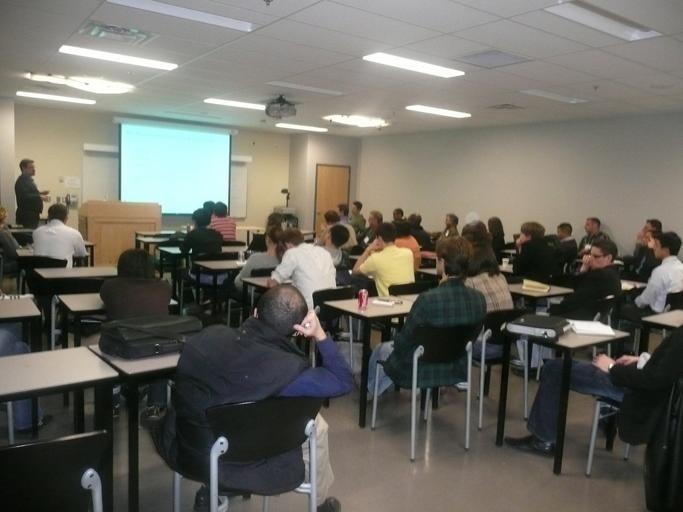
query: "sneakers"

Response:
[193,485,228,511]
[354,373,374,404]
[112,403,121,418]
[317,497,341,512]
[141,406,160,420]
[15,416,53,436]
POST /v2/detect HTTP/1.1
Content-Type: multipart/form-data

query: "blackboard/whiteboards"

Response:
[82,143,253,219]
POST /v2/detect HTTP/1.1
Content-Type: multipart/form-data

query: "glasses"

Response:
[590,254,606,258]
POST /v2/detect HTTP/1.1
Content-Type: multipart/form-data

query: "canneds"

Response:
[357,288,368,312]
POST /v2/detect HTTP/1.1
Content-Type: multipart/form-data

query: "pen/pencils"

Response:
[304,305,320,329]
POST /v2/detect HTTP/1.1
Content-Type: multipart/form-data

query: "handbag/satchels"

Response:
[100,314,203,358]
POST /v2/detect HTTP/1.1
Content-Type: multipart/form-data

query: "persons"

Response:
[360,234,489,403]
[146,281,358,511]
[176,202,682,393]
[0,325,55,437]
[1,205,25,279]
[14,158,50,230]
[31,202,92,271]
[502,324,682,456]
[101,246,169,426]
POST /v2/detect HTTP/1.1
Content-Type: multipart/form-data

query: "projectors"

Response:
[266,103,297,120]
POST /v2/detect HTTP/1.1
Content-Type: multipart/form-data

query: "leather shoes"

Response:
[599,416,616,431]
[505,435,555,457]
